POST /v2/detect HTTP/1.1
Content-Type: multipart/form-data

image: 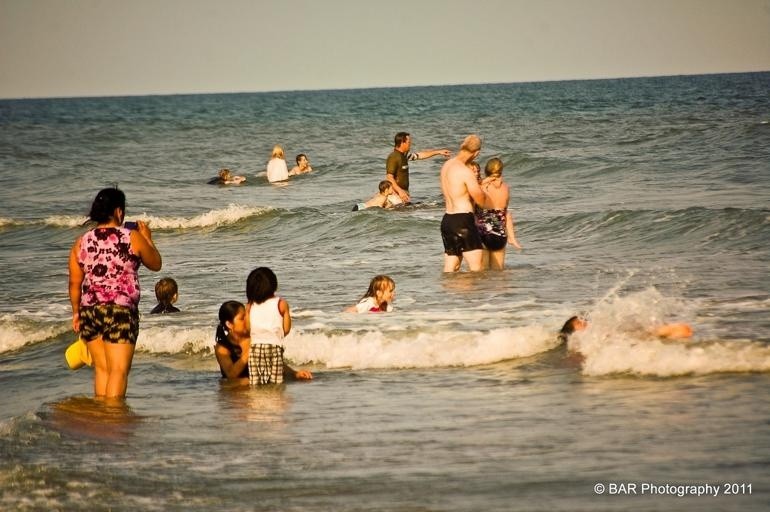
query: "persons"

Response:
[264,145,290,182]
[206,168,248,185]
[350,180,394,212]
[437,135,487,273]
[341,274,396,311]
[215,297,315,388]
[385,133,451,208]
[148,277,181,314]
[241,266,291,385]
[68,186,163,407]
[559,315,696,339]
[466,160,523,251]
[474,158,510,271]
[288,155,315,176]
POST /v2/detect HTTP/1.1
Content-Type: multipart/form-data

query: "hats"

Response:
[65,333,94,370]
